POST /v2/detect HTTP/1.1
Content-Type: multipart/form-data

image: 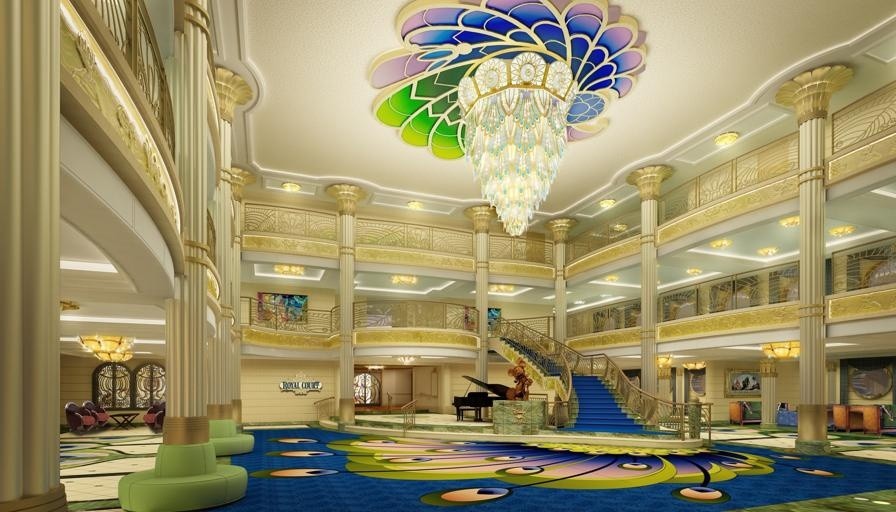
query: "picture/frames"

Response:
[723,367,761,399]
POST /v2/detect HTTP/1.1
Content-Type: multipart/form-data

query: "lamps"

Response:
[761,341,802,360]
[655,356,672,370]
[680,360,707,371]
[714,131,739,145]
[598,199,616,210]
[612,224,628,232]
[407,201,424,211]
[280,182,302,194]
[59,301,135,363]
[273,264,305,275]
[456,51,580,238]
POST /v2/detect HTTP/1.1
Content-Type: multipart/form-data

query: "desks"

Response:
[832,404,881,437]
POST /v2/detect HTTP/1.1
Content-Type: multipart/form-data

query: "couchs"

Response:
[774,410,798,427]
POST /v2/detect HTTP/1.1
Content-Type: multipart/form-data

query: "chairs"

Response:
[64,399,166,435]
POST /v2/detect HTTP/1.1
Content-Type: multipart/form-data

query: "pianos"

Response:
[454,375,511,422]
